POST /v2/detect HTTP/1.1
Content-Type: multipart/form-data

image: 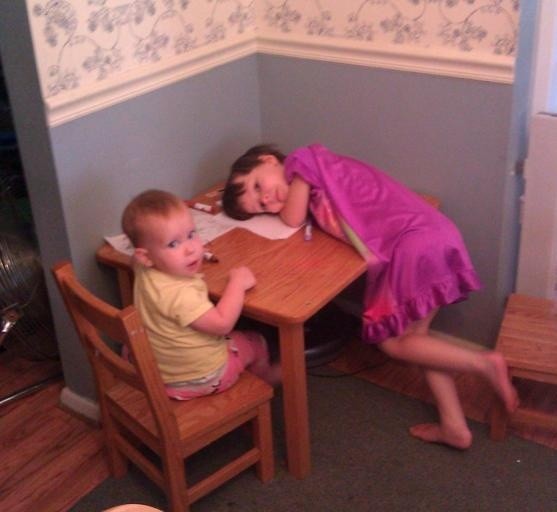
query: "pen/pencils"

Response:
[205,251,219,263]
[184,200,217,213]
[304,218,313,241]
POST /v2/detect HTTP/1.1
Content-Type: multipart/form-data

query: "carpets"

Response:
[67,360,553,511]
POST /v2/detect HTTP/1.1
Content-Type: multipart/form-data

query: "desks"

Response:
[93,163,445,479]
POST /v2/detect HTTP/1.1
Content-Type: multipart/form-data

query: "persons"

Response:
[120,188,282,401]
[225,143,521,452]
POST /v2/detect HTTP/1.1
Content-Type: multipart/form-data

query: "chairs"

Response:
[49,261,278,512]
[482,290,557,444]
